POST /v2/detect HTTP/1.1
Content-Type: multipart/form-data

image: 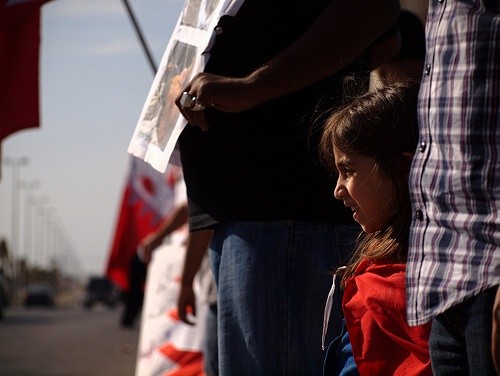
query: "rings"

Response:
[180,92,195,108]
[192,97,206,111]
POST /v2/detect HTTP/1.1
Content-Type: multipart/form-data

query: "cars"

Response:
[22,283,56,310]
[83,275,116,311]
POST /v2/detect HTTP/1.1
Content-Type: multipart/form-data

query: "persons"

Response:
[175,0,426,376]
[404,1,499,376]
[321,78,436,375]
[136,172,216,375]
